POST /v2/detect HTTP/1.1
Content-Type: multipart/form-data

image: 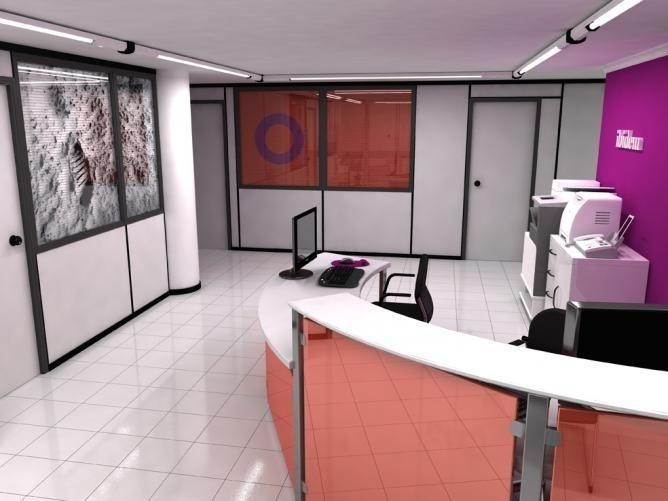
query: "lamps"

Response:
[512,0,644,79]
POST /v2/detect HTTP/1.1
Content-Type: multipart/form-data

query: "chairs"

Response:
[509,308,565,354]
[372,254,435,323]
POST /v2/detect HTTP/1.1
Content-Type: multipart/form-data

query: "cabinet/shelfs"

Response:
[544,234,649,311]
